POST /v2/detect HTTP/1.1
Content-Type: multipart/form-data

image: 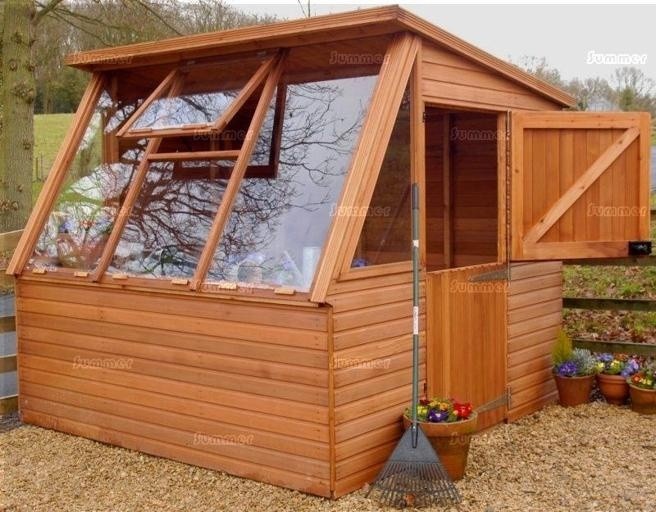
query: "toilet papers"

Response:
[302,246,319,292]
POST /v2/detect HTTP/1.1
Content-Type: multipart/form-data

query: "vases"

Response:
[629,384,656,414]
[552,372,597,407]
[596,373,629,406]
[403,409,477,481]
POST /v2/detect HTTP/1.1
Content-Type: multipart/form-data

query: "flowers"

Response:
[594,352,646,377]
[625,356,656,389]
[553,347,597,377]
[406,395,474,422]
